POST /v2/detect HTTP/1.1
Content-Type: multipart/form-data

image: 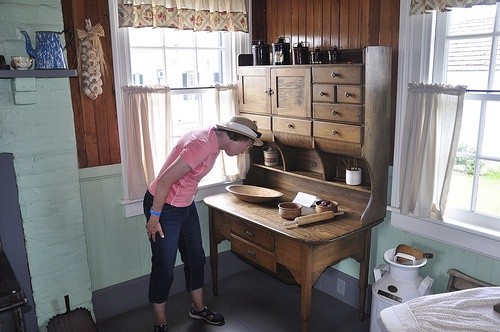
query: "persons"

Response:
[143,116,263,332]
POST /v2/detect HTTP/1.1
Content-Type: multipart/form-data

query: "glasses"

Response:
[241,136,252,150]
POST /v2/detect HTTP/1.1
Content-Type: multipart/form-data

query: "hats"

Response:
[216,116,263,146]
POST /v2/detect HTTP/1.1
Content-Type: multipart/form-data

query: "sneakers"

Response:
[153,320,171,332]
[188,303,224,324]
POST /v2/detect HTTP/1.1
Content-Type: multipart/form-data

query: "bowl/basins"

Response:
[315,200,339,213]
[278,202,303,219]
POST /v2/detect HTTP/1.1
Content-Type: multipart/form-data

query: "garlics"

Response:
[82,36,102,100]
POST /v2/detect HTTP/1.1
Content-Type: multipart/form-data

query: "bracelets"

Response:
[149,208,161,217]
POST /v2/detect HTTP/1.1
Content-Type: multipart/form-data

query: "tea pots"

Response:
[20,29,74,70]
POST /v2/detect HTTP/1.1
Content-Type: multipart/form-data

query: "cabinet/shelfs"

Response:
[203,46,392,332]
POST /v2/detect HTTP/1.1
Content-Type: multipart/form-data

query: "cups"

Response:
[10,56,33,70]
[346,167,361,185]
